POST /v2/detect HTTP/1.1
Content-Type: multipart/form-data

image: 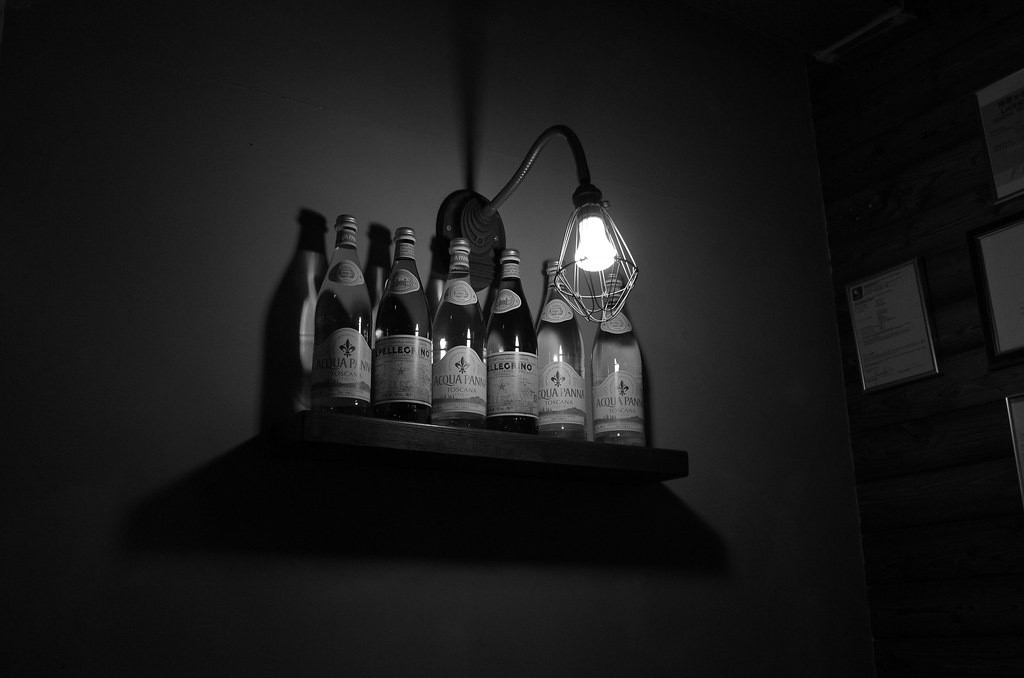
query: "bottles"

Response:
[432,238,487,428]
[486,249,539,433]
[374,228,432,424]
[536,261,586,438]
[311,215,371,416]
[590,272,644,446]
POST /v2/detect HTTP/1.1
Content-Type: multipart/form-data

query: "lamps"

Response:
[436,124,638,323]
